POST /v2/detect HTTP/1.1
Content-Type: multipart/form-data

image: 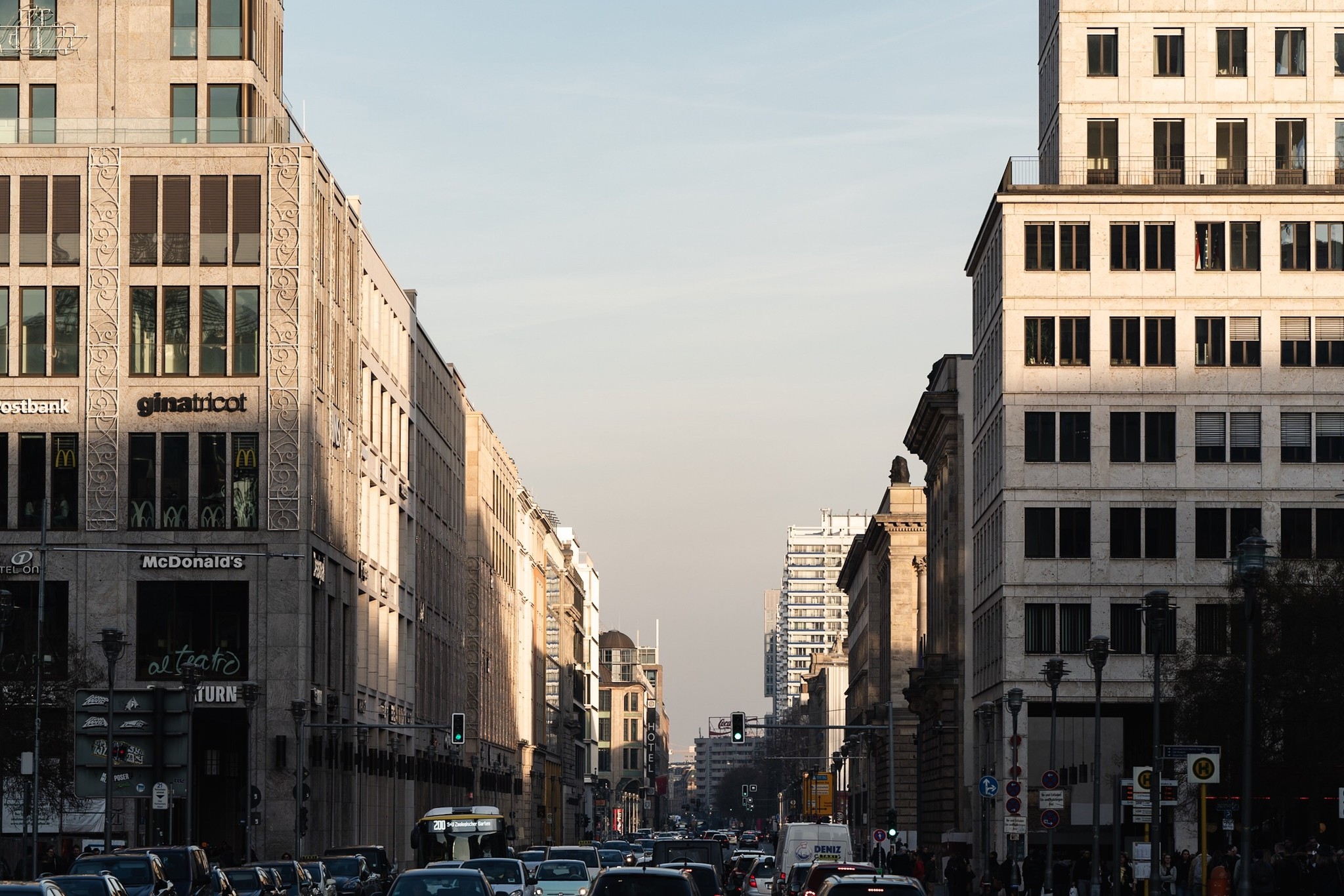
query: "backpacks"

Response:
[1192,856,1210,889]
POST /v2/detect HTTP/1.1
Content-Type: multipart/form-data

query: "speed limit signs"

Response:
[837,811,844,821]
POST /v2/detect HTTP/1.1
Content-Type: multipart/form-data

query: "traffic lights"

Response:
[886,808,898,840]
[741,784,757,811]
[709,804,713,815]
[731,712,745,744]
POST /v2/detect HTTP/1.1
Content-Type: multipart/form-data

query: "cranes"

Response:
[668,744,696,763]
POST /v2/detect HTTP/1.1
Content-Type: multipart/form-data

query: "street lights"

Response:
[174,660,205,845]
[1136,591,1182,896]
[834,732,871,861]
[91,627,132,855]
[1038,659,1074,894]
[506,764,523,847]
[974,701,999,896]
[447,749,460,808]
[491,760,504,808]
[423,745,441,810]
[386,737,405,864]
[466,754,483,807]
[782,765,820,824]
[451,713,466,744]
[1082,634,1117,896]
[324,718,347,850]
[1002,688,1030,896]
[235,680,268,866]
[286,699,312,861]
[1223,529,1275,896]
[352,727,373,845]
[526,768,640,850]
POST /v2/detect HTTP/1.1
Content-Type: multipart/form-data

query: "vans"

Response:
[771,822,853,896]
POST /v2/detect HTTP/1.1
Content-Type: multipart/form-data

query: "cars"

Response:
[777,857,927,896]
[586,822,776,896]
[386,841,603,896]
[0,845,397,896]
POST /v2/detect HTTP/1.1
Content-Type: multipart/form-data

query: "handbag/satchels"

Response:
[1069,886,1078,896]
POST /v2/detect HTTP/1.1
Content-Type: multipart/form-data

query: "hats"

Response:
[1121,851,1130,862]
[900,846,908,851]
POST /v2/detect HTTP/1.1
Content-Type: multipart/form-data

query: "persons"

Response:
[1107,851,1138,896]
[896,838,903,851]
[218,841,234,866]
[1161,836,1344,896]
[988,848,1093,896]
[586,830,593,840]
[855,843,871,862]
[14,844,100,882]
[944,851,972,896]
[886,843,934,891]
[281,853,292,860]
[872,842,886,868]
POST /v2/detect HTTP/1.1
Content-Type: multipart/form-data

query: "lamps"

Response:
[1079,715,1087,783]
[1060,716,1068,785]
[1069,716,1077,784]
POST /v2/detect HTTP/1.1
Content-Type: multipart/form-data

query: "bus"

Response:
[411,805,510,869]
